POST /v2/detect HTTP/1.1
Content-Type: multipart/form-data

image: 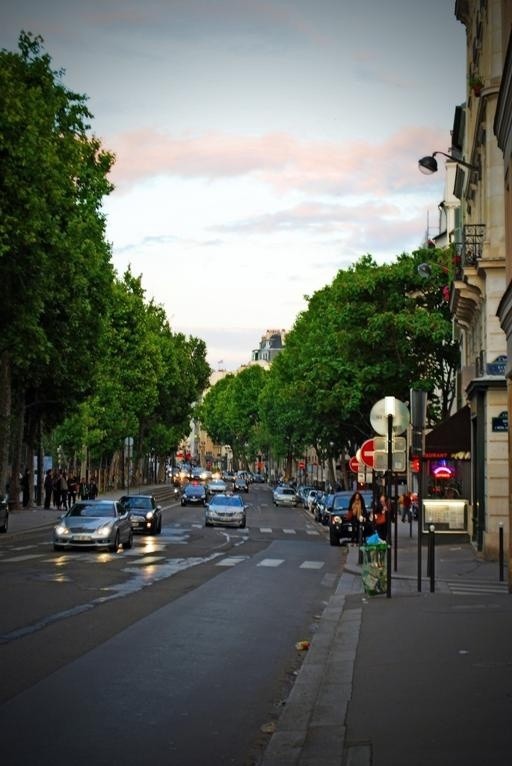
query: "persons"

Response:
[399,491,418,523]
[376,495,391,541]
[348,492,370,547]
[5,466,98,512]
[171,475,183,497]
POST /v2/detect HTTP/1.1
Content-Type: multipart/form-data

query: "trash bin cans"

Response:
[360,546,389,596]
[0,502,10,535]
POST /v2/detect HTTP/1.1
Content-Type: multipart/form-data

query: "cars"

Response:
[209,479,227,494]
[54,500,133,551]
[299,486,375,546]
[119,495,162,535]
[273,486,298,507]
[205,494,249,528]
[234,480,248,493]
[181,480,207,507]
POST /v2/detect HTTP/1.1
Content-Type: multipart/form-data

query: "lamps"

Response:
[416,261,450,279]
[418,150,482,181]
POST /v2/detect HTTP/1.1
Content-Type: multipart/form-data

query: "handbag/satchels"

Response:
[374,513,386,524]
[345,511,354,519]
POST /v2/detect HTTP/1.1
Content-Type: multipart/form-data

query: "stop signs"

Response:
[360,439,375,468]
[348,456,358,473]
[208,463,211,467]
[299,461,305,469]
